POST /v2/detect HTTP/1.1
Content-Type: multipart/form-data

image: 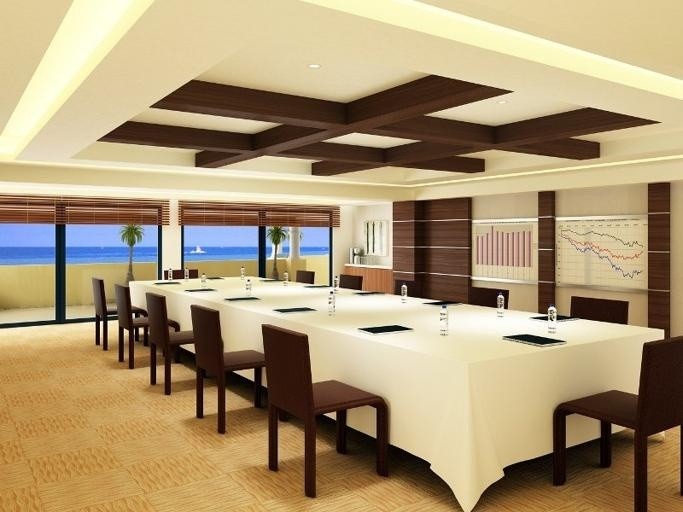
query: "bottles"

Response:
[246,280,251,296]
[283,270,289,287]
[440,305,448,336]
[201,272,207,287]
[240,266,245,280]
[168,268,173,280]
[497,291,504,317]
[401,283,407,303]
[334,276,340,294]
[184,268,190,279]
[548,304,557,335]
[329,291,336,317]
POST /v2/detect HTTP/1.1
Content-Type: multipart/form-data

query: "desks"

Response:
[129,277,665,512]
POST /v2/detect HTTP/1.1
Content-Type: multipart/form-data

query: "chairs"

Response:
[469,287,509,309]
[91,277,148,350]
[296,271,315,284]
[340,275,363,290]
[190,304,265,433]
[552,334,682,512]
[164,269,198,279]
[146,292,206,394]
[570,295,629,325]
[262,323,388,498]
[395,280,423,296]
[115,282,180,368]
